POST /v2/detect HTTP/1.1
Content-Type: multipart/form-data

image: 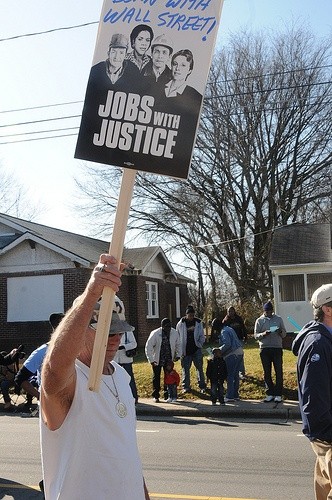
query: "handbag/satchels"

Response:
[125,332,137,357]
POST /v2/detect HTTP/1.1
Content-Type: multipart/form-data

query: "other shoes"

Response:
[155,399,159,403]
[166,397,177,403]
[181,388,191,393]
[224,397,240,402]
[135,396,138,404]
[212,402,215,405]
[4,401,11,409]
[274,395,281,402]
[164,395,168,400]
[264,395,274,402]
[200,389,206,394]
[220,403,225,406]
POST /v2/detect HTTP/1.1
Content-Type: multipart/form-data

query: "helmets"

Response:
[150,33,173,55]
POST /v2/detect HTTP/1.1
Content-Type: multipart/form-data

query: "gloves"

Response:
[118,346,125,350]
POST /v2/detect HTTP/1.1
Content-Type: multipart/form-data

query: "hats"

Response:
[109,33,129,51]
[263,301,273,311]
[311,284,332,307]
[73,293,135,335]
[49,312,65,327]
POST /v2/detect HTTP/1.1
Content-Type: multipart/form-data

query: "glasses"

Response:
[109,333,124,337]
[266,312,272,313]
[52,322,60,329]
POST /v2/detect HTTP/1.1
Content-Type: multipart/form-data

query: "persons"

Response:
[112,331,138,403]
[39,251,149,500]
[13,341,51,401]
[211,318,244,403]
[176,305,207,393]
[1,348,18,412]
[145,318,182,402]
[222,305,246,377]
[254,301,287,402]
[205,348,228,406]
[164,360,180,402]
[292,283,332,500]
[88,24,203,106]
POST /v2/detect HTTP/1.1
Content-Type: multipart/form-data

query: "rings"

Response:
[99,264,105,272]
